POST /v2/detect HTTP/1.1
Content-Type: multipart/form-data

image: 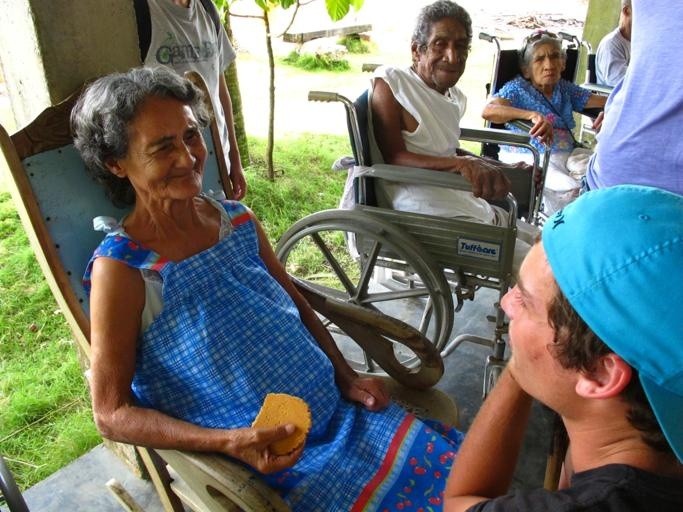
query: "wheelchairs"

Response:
[578,39,614,147]
[477,31,603,230]
[270,64,540,404]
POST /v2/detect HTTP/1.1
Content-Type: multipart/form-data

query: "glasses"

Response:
[522,31,562,59]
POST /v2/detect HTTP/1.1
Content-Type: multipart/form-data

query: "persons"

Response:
[479,29,610,208]
[576,1,682,211]
[127,0,248,218]
[432,182,682,511]
[587,2,631,89]
[65,61,469,511]
[359,0,546,280]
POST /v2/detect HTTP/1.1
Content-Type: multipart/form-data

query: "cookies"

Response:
[252,392,312,456]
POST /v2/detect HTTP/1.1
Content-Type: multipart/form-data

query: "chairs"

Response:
[0,69,459,512]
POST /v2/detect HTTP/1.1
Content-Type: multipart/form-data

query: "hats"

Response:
[542,184,683,464]
